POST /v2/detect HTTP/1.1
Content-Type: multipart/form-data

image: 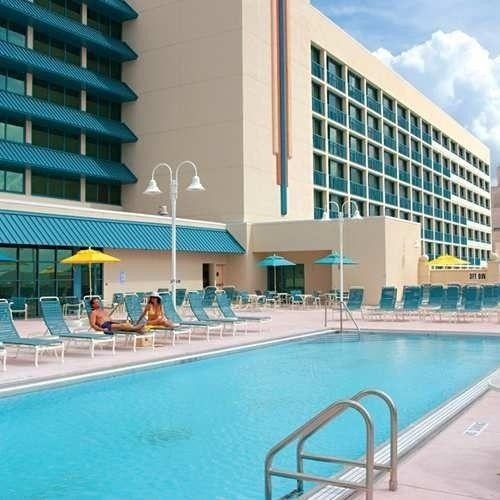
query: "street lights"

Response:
[141,159,207,311]
[320,198,364,307]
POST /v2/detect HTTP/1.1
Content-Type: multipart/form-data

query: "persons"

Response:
[135,292,180,327]
[90,298,148,334]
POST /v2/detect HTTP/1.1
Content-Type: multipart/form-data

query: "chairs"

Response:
[215,289,273,337]
[39,295,115,358]
[73,295,156,352]
[330,287,365,321]
[1,298,65,369]
[240,284,345,312]
[159,291,223,341]
[122,293,193,347]
[182,290,249,336]
[366,283,500,323]
[0,285,240,319]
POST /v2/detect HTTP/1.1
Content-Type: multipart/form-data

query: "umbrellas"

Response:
[37,266,64,275]
[313,248,360,294]
[426,255,471,269]
[60,246,120,301]
[256,251,296,295]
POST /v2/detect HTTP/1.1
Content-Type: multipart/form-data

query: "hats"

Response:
[146,292,161,298]
